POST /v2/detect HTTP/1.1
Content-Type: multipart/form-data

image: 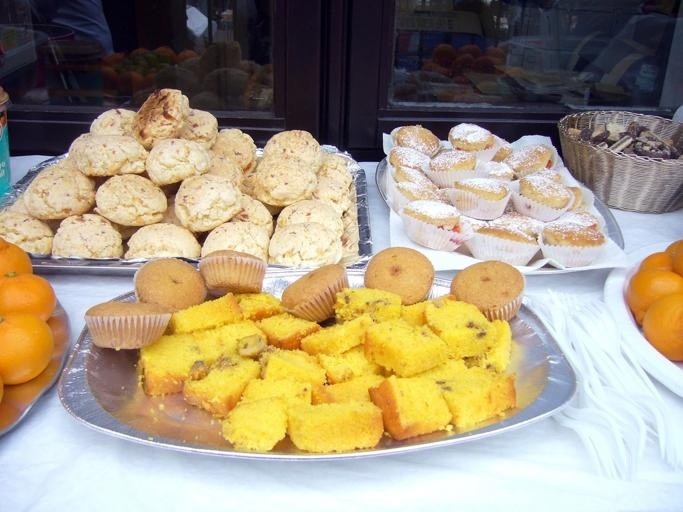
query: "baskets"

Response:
[558,110,683,213]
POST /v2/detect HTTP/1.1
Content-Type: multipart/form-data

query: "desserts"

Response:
[1,92,354,271]
[82,248,525,454]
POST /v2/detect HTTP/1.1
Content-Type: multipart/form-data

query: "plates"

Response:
[0,291,73,442]
[54,268,581,463]
[372,149,625,276]
[603,243,681,403]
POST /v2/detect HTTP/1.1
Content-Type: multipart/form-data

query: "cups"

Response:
[0,85,17,202]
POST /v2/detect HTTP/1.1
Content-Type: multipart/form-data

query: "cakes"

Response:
[382,121,608,271]
[394,70,484,105]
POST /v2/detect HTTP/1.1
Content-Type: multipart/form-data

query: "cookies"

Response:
[568,121,682,161]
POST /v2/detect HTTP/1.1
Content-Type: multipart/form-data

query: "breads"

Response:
[157,42,272,111]
